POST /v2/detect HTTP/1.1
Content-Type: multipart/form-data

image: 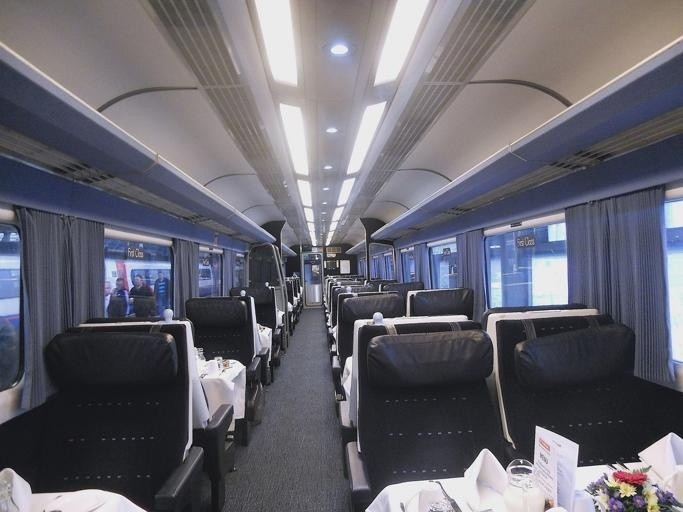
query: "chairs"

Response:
[0,287,286,510]
[285,275,306,335]
[323,276,540,510]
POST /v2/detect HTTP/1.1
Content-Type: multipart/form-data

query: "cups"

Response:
[367,322,374,325]
[326,275,335,282]
[193,347,232,376]
[372,311,383,325]
[336,282,341,287]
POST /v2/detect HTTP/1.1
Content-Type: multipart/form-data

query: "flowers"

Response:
[584,470,679,512]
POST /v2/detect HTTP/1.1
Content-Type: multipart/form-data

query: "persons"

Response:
[111,278,134,316]
[129,274,157,316]
[104,281,128,318]
[154,271,170,315]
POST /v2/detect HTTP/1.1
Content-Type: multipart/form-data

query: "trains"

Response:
[0,225,246,370]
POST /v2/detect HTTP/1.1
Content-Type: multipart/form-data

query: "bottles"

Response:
[346,287,351,293]
[239,290,245,296]
[264,282,268,286]
[162,308,172,322]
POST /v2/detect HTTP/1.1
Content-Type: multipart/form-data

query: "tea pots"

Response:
[502,458,545,512]
